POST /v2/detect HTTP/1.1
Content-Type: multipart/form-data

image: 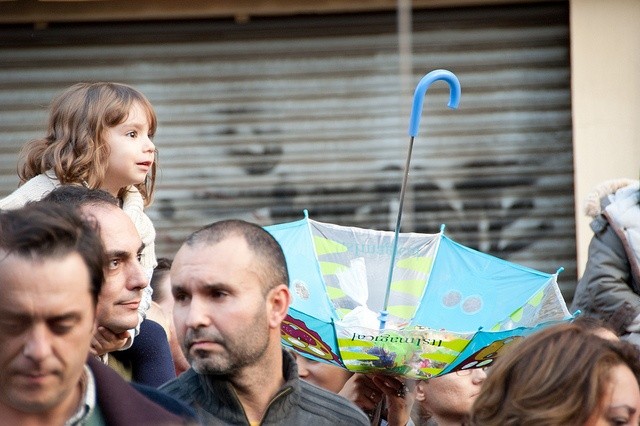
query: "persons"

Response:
[150,256,191,375]
[1,80,178,389]
[567,178,640,344]
[25,184,148,383]
[571,315,620,341]
[412,367,487,426]
[469,322,640,426]
[285,346,417,426]
[153,218,374,426]
[1,203,206,426]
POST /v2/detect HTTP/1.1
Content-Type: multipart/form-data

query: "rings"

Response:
[370,391,376,399]
[396,388,405,398]
[400,382,407,387]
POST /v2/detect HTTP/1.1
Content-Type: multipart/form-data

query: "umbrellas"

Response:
[264,67,583,426]
